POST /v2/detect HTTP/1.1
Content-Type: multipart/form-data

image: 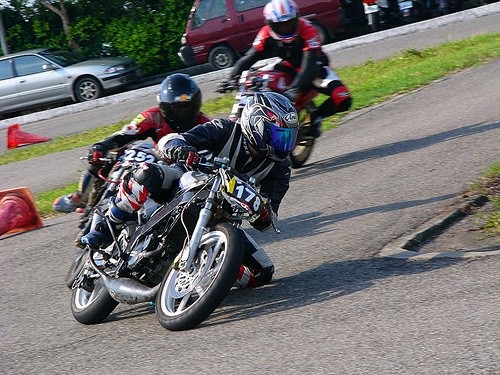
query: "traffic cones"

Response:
[5,123,50,151]
[0,186,44,236]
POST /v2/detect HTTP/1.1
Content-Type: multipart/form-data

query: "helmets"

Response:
[263,0,299,44]
[155,73,202,132]
[240,92,300,161]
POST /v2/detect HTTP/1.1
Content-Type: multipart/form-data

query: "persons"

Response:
[82,93,300,291]
[218,0,352,137]
[51,74,212,278]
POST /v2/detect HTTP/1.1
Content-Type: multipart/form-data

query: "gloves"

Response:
[162,138,200,166]
[283,87,301,102]
[250,204,277,233]
[87,141,107,164]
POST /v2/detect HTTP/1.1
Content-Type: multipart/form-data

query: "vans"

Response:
[177,0,351,72]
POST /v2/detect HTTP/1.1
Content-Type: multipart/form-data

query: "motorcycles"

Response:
[70,55,326,332]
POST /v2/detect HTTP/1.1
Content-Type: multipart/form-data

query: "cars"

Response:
[0,45,144,116]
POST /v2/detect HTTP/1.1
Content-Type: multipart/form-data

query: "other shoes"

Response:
[52,191,80,213]
[81,228,104,250]
[310,108,322,137]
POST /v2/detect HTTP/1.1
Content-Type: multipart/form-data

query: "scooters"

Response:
[359,0,455,33]
